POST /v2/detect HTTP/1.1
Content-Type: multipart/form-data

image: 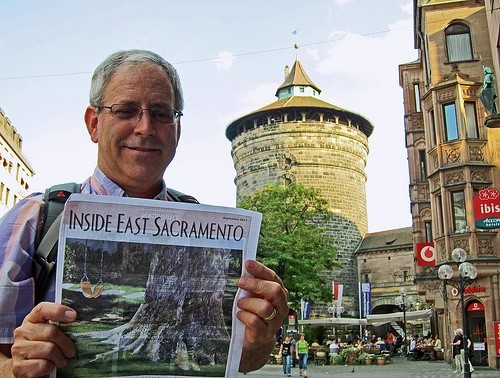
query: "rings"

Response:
[264,306,278,322]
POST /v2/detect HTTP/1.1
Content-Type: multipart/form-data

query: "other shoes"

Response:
[300,371,303,377]
[288,374,291,377]
[303,372,307,378]
[284,374,287,376]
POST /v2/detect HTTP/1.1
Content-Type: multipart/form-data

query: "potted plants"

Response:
[332,347,386,366]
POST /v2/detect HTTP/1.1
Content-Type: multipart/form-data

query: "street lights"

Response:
[328,299,345,344]
[437,247,478,378]
[394,287,415,357]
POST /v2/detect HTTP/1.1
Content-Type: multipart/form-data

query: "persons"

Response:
[278,330,443,378]
[449,328,475,373]
[0,50,290,378]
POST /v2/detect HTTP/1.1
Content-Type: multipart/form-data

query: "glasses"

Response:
[92,103,184,125]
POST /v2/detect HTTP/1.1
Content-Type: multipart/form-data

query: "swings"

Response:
[81,240,105,298]
